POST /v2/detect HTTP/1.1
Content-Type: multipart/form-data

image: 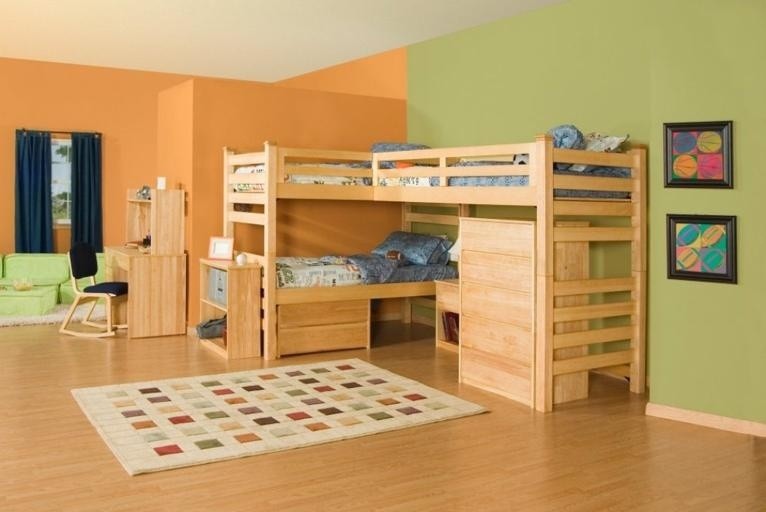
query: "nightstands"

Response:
[435,280,461,352]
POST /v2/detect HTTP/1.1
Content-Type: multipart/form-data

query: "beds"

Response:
[222,142,648,415]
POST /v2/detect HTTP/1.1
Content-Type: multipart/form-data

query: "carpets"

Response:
[0,302,105,328]
[70,357,492,476]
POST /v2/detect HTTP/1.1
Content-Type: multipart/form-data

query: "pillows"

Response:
[373,229,449,264]
[571,132,627,173]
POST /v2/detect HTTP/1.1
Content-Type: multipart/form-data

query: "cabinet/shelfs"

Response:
[459,213,590,414]
[195,259,260,360]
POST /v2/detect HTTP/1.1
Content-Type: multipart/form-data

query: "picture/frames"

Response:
[664,122,736,283]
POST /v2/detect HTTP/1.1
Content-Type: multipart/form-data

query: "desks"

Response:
[103,185,185,339]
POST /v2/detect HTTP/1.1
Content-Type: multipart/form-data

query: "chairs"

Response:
[1,254,102,314]
[59,242,127,338]
